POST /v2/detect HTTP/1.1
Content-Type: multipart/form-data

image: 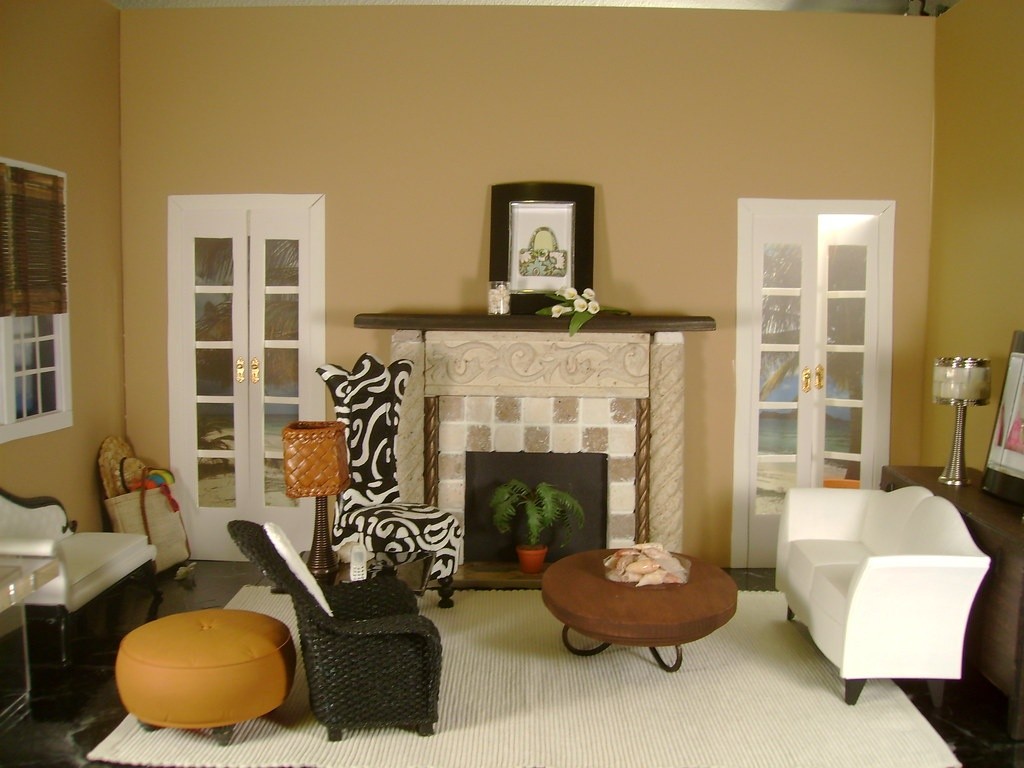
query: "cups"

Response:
[488,281,511,315]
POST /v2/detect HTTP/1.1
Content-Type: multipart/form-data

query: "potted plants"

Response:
[489,479,586,573]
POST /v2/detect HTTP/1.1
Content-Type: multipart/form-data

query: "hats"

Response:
[99,435,175,498]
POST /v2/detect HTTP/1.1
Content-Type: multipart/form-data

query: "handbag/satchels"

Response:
[104,466,191,576]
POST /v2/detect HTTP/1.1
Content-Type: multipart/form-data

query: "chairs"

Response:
[0,488,163,678]
[227,521,442,741]
[775,487,991,706]
[316,351,464,608]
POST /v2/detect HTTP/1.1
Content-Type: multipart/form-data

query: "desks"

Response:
[542,548,737,672]
[1,559,59,736]
[882,466,1024,740]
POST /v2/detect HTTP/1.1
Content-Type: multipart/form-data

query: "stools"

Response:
[116,608,297,746]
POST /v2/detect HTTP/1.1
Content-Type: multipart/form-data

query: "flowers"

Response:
[535,286,630,337]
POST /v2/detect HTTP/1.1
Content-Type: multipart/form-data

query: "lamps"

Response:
[932,357,991,485]
[282,422,351,574]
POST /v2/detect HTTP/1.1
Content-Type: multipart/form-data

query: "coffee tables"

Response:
[270,551,436,595]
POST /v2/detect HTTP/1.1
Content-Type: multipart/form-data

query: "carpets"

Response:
[87,586,964,768]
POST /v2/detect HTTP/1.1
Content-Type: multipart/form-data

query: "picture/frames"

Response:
[489,181,594,314]
